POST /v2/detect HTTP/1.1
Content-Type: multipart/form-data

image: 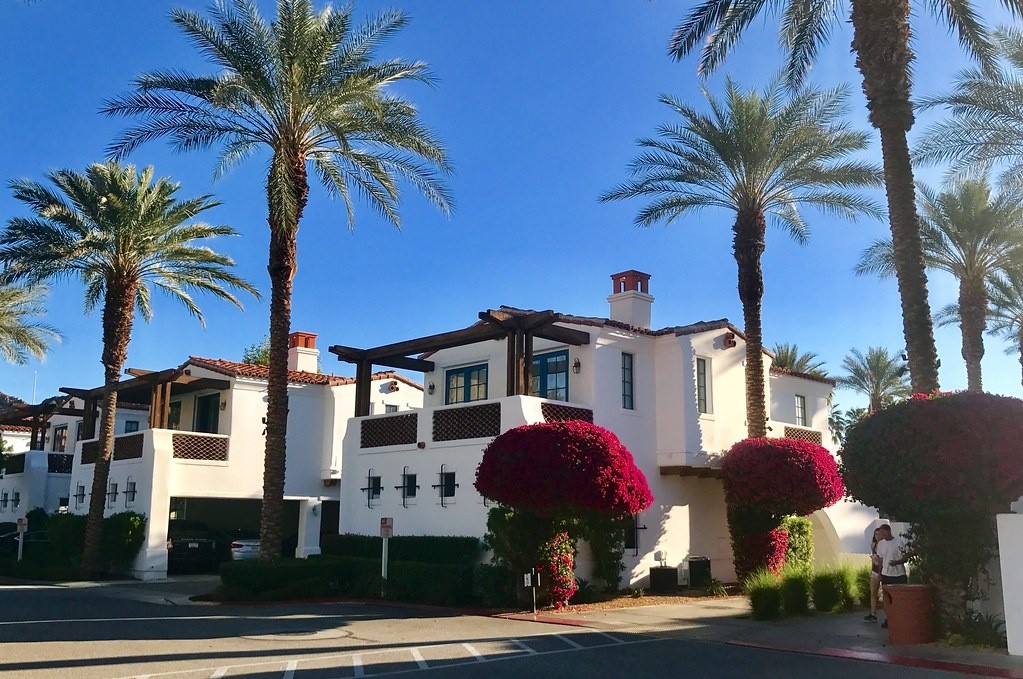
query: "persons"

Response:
[863,524,909,628]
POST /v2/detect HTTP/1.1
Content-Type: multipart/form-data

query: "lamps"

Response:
[428,380,435,395]
[219,399,226,411]
[572,358,581,373]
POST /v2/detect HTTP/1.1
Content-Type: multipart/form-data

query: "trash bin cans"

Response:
[683,555,712,590]
[882,583,935,645]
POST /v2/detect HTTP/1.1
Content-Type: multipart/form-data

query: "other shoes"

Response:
[864,613,877,620]
[881,619,888,628]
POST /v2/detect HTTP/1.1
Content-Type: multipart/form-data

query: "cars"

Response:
[166,517,225,576]
[230,529,266,562]
